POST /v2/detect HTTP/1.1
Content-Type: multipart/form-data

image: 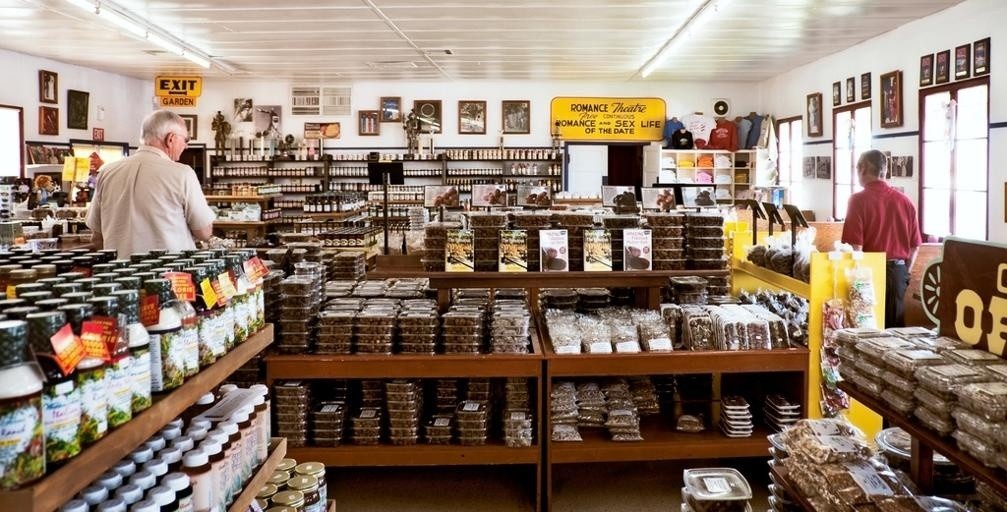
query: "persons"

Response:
[28,174,54,210]
[86,168,100,202]
[85,109,217,259]
[839,148,922,328]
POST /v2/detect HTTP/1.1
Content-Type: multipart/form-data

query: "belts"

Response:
[886,259,907,267]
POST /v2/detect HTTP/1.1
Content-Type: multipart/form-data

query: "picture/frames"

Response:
[502,99,530,134]
[919,54,933,86]
[935,49,950,84]
[178,113,198,140]
[955,44,971,81]
[973,36,991,76]
[847,77,855,103]
[458,100,486,133]
[414,99,442,133]
[39,70,58,104]
[881,69,900,127]
[832,82,841,106]
[67,89,89,130]
[358,110,380,135]
[380,96,402,122]
[807,92,823,137]
[39,106,60,134]
[861,72,871,100]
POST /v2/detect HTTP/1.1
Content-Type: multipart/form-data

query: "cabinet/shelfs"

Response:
[0,176,337,512]
[765,236,1007,512]
[208,138,815,510]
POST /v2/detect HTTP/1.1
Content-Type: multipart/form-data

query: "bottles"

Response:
[0,176,36,225]
[1,244,327,512]
[211,137,563,249]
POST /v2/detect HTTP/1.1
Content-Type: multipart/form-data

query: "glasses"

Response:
[170,132,191,143]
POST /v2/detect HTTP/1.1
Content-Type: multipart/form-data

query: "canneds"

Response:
[212,166,443,248]
[255,458,328,512]
[447,168,503,176]
[446,149,556,160]
